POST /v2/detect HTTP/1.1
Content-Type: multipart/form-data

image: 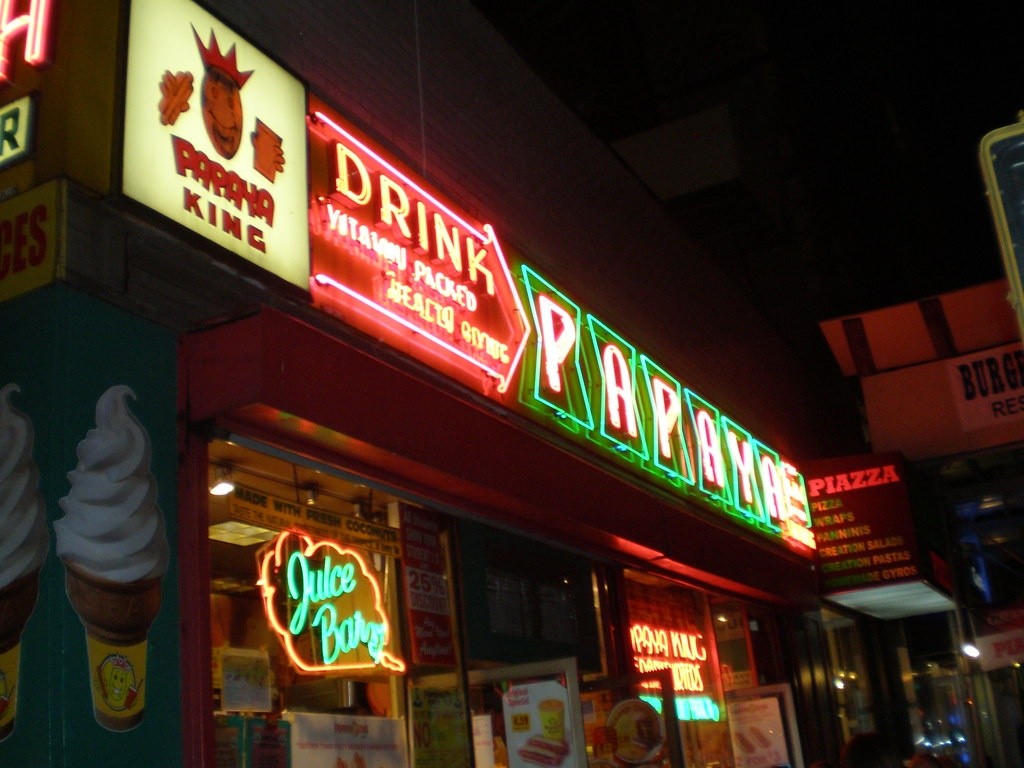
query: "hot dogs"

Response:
[734,726,771,753]
[517,735,570,768]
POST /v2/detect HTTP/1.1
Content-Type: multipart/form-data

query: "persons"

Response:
[840,732,905,768]
[811,760,843,768]
[908,750,943,768]
[937,754,962,768]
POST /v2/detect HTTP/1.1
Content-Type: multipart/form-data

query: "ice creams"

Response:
[0,382,50,743]
[51,383,172,731]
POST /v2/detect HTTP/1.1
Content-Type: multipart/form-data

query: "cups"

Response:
[538,700,565,741]
